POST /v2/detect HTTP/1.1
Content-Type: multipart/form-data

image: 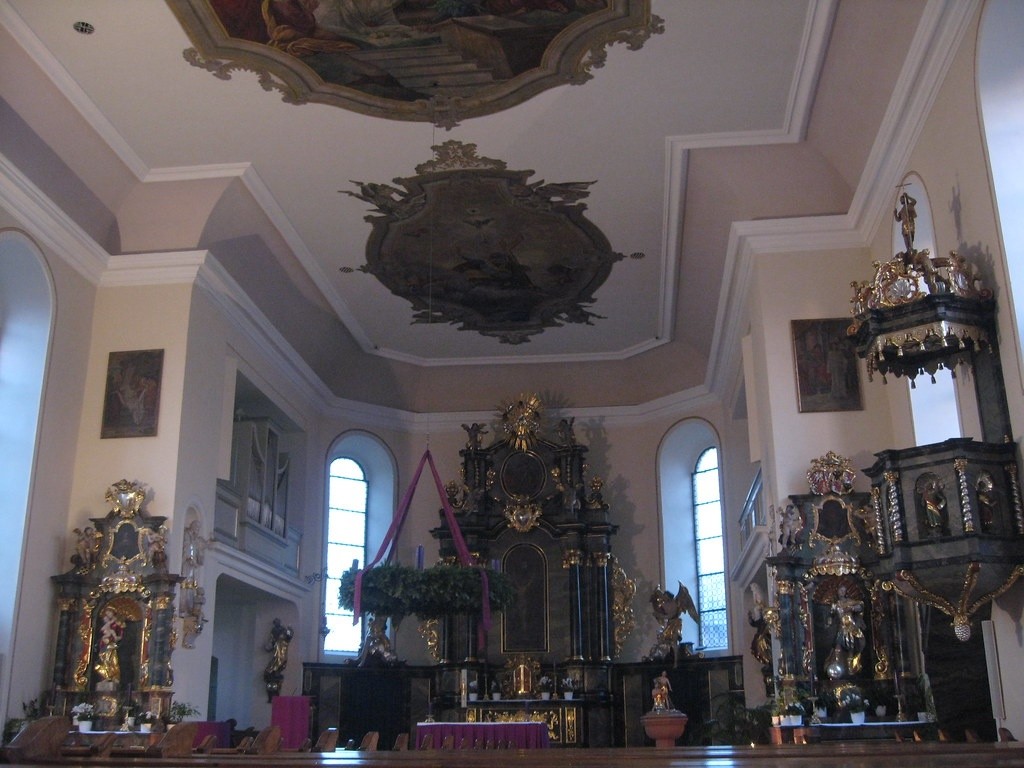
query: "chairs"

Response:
[91,722,515,758]
[801,725,1021,741]
[1,716,70,763]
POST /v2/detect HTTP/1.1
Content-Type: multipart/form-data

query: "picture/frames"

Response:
[100,348,165,440]
[790,317,863,412]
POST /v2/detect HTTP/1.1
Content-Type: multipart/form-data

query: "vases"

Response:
[73,714,80,728]
[564,691,573,700]
[492,692,501,701]
[469,692,478,702]
[851,711,866,724]
[139,722,152,733]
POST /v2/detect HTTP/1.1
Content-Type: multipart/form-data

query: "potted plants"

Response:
[813,694,829,717]
[873,700,886,717]
[76,713,95,733]
[907,673,935,721]
[163,713,177,731]
[771,704,804,726]
[128,710,135,728]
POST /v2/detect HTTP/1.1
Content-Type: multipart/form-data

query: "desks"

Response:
[416,722,550,753]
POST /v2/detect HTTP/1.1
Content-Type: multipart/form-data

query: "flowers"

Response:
[561,677,575,691]
[842,694,870,713]
[541,691,550,700]
[468,681,478,693]
[534,676,553,692]
[137,712,156,724]
[71,703,94,714]
[490,682,501,693]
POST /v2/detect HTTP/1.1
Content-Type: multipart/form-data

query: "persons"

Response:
[192,587,208,629]
[183,519,210,586]
[654,591,685,669]
[977,480,995,529]
[767,504,778,556]
[552,416,578,444]
[462,422,490,451]
[920,480,944,528]
[777,502,800,549]
[264,618,294,676]
[750,581,767,622]
[653,670,673,711]
[94,605,126,686]
[99,615,122,643]
[893,191,920,250]
[75,527,99,565]
[852,503,879,543]
[145,525,169,561]
[824,585,865,676]
